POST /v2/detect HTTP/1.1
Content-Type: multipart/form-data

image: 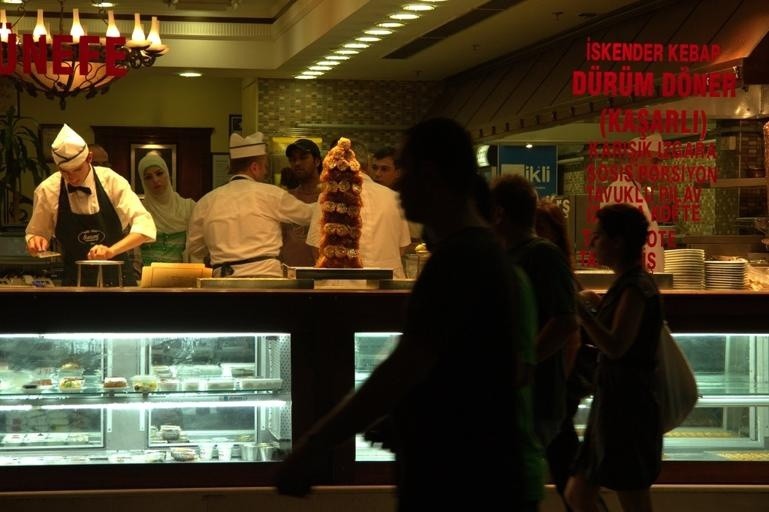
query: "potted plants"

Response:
[0,103,50,263]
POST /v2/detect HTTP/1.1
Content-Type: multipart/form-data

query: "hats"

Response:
[229,132,266,159]
[286,139,320,156]
[51,123,89,170]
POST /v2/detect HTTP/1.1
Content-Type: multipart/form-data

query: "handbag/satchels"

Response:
[655,321,697,434]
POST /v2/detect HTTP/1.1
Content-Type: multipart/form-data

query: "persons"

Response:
[23,123,159,286]
[563,201,676,512]
[279,138,328,271]
[533,198,576,270]
[368,145,402,188]
[484,170,584,512]
[130,150,209,276]
[305,139,412,287]
[186,132,330,280]
[271,117,546,512]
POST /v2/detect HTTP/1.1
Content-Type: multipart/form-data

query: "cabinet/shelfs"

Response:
[0,282,768,493]
[677,117,769,261]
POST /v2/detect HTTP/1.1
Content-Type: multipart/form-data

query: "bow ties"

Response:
[68,183,91,194]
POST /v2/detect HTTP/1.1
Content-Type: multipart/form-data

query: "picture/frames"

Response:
[229,113,242,137]
[129,142,178,200]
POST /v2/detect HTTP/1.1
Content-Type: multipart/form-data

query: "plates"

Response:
[704,259,749,290]
[662,248,705,290]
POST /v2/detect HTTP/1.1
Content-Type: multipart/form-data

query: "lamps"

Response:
[0,0,169,112]
[292,3,436,80]
